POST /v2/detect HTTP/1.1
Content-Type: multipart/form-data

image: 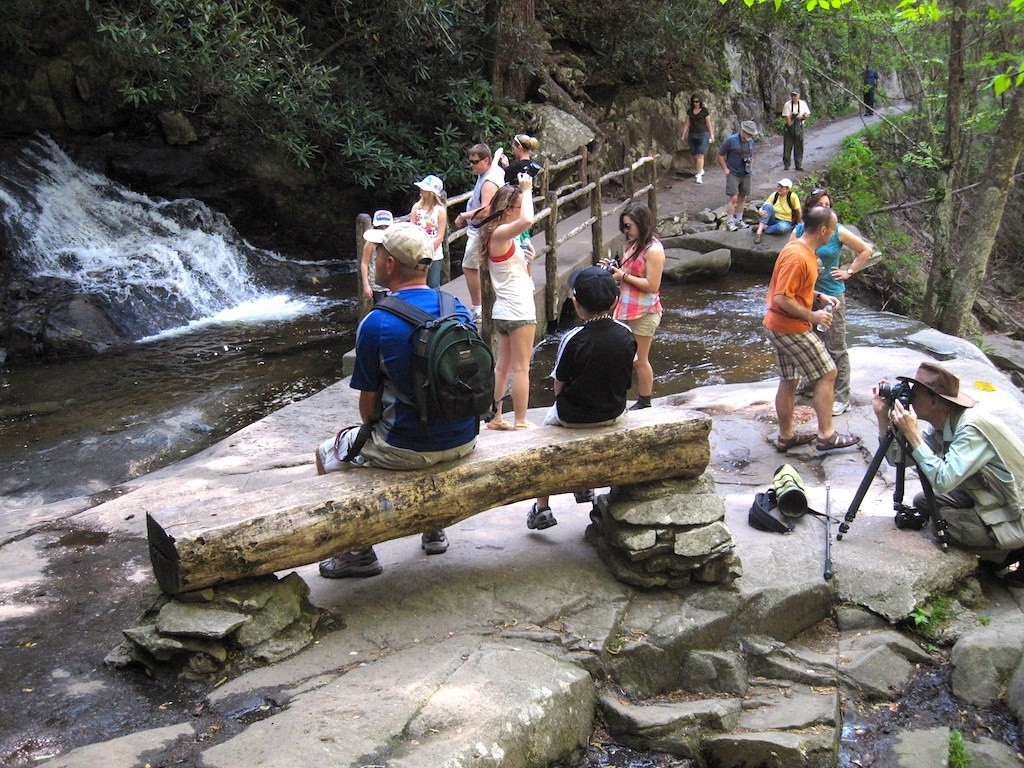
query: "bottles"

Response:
[521,239,529,263]
[817,302,833,332]
[817,257,826,280]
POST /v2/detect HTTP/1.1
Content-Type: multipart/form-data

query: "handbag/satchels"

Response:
[788,100,802,138]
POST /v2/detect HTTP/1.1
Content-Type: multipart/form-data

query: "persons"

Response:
[680,93,714,183]
[360,210,394,306]
[316,222,481,579]
[781,88,811,171]
[453,134,540,431]
[595,202,665,410]
[717,120,758,231]
[410,174,447,291]
[752,178,803,244]
[762,188,873,453]
[862,65,878,116]
[527,266,639,530]
[871,362,1024,589]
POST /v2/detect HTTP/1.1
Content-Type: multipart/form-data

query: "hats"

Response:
[567,264,618,311]
[778,177,792,190]
[414,175,444,198]
[789,88,800,95]
[363,222,435,271]
[372,210,393,227]
[741,120,758,135]
[895,361,975,408]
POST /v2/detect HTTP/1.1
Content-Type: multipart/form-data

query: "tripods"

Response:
[836,405,948,552]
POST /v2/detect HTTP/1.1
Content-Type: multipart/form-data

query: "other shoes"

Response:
[984,547,1024,588]
[629,402,651,412]
[468,309,482,324]
[752,226,765,244]
[694,170,705,184]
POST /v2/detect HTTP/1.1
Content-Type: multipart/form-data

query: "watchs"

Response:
[847,267,855,276]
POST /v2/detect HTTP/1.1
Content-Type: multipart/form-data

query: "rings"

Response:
[893,414,897,419]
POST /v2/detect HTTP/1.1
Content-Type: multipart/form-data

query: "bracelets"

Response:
[816,291,825,303]
[625,274,629,281]
[622,272,626,280]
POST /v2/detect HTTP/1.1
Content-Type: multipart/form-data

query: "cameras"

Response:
[515,161,540,184]
[877,380,914,409]
[600,258,619,274]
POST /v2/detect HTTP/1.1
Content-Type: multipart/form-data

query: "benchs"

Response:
[104,408,742,687]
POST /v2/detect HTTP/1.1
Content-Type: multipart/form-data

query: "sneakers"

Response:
[726,220,738,232]
[318,546,383,579]
[831,400,851,415]
[422,527,450,556]
[736,220,749,229]
[527,502,558,531]
[573,488,596,503]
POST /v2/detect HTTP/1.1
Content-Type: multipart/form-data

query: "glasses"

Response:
[810,188,825,196]
[467,134,522,165]
[620,221,635,232]
[692,100,700,104]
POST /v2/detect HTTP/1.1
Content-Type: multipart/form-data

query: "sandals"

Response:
[486,419,534,433]
[777,428,862,452]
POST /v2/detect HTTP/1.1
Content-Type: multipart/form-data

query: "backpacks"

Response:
[370,290,496,428]
